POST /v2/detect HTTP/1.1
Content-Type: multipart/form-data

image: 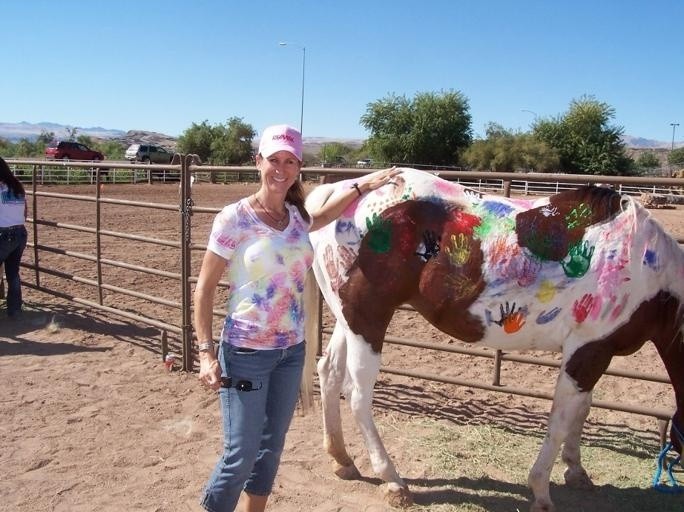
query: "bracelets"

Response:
[351,183,361,196]
[199,343,214,351]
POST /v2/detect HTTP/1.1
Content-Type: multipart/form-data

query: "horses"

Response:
[296,166,684,511]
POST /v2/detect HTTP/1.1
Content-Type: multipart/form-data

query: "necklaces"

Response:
[253,193,287,226]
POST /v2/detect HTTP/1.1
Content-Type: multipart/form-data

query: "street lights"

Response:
[279,42,308,184]
[520,109,538,120]
[669,122,680,151]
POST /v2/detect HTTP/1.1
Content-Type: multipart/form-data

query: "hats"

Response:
[255,124,305,162]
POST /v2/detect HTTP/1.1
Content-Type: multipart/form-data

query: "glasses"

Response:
[217,374,264,394]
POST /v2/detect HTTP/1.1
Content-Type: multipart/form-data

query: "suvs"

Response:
[123,143,174,164]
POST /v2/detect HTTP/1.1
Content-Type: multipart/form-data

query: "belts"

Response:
[0,224,25,231]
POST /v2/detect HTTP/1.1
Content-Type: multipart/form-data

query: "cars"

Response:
[315,156,347,167]
[355,157,375,167]
[43,139,105,163]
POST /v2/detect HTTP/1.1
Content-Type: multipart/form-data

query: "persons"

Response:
[193,124,403,512]
[0,157,28,319]
